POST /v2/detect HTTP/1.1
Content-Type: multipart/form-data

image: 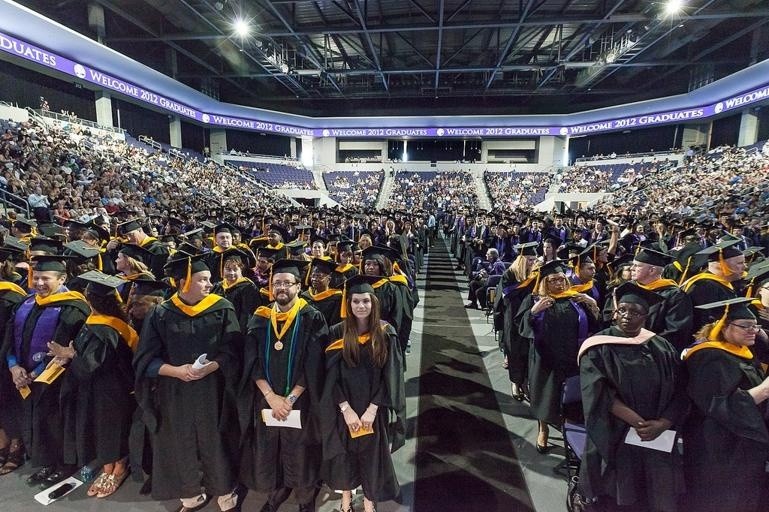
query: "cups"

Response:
[81,465,93,482]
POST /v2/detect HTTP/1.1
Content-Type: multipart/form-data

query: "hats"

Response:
[0,207,424,293]
[512,239,768,281]
[612,281,663,312]
[696,294,760,321]
[455,208,746,233]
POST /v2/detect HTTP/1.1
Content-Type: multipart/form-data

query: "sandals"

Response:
[362,492,376,511]
[503,356,509,369]
[1,446,27,475]
[338,493,354,512]
[85,470,130,499]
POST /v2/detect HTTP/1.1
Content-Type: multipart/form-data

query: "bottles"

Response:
[405,338,411,354]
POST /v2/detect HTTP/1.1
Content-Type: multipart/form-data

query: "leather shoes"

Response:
[260,492,288,512]
[512,380,530,402]
[464,302,478,308]
[176,485,208,512]
[216,483,248,512]
[29,462,67,488]
[299,488,320,512]
[536,425,550,453]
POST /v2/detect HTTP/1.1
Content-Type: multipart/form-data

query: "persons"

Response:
[1,98,421,512]
[416,168,474,266]
[464,139,768,512]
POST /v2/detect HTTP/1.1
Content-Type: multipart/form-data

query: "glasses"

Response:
[618,307,646,316]
[726,320,763,330]
[548,277,567,283]
